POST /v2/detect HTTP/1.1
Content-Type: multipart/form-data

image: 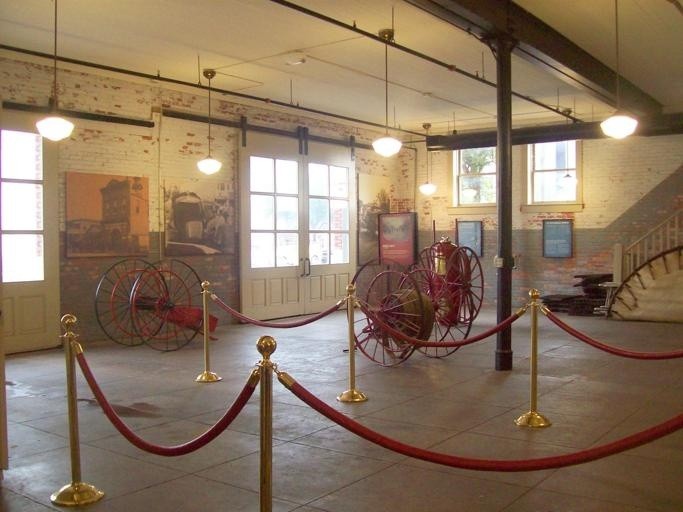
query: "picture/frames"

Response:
[541,217,574,259]
[377,212,418,267]
[456,220,483,258]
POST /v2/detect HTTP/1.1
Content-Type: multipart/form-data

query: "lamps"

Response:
[372,28,438,196]
[196,67,223,175]
[600,0,638,142]
[32,0,76,142]
